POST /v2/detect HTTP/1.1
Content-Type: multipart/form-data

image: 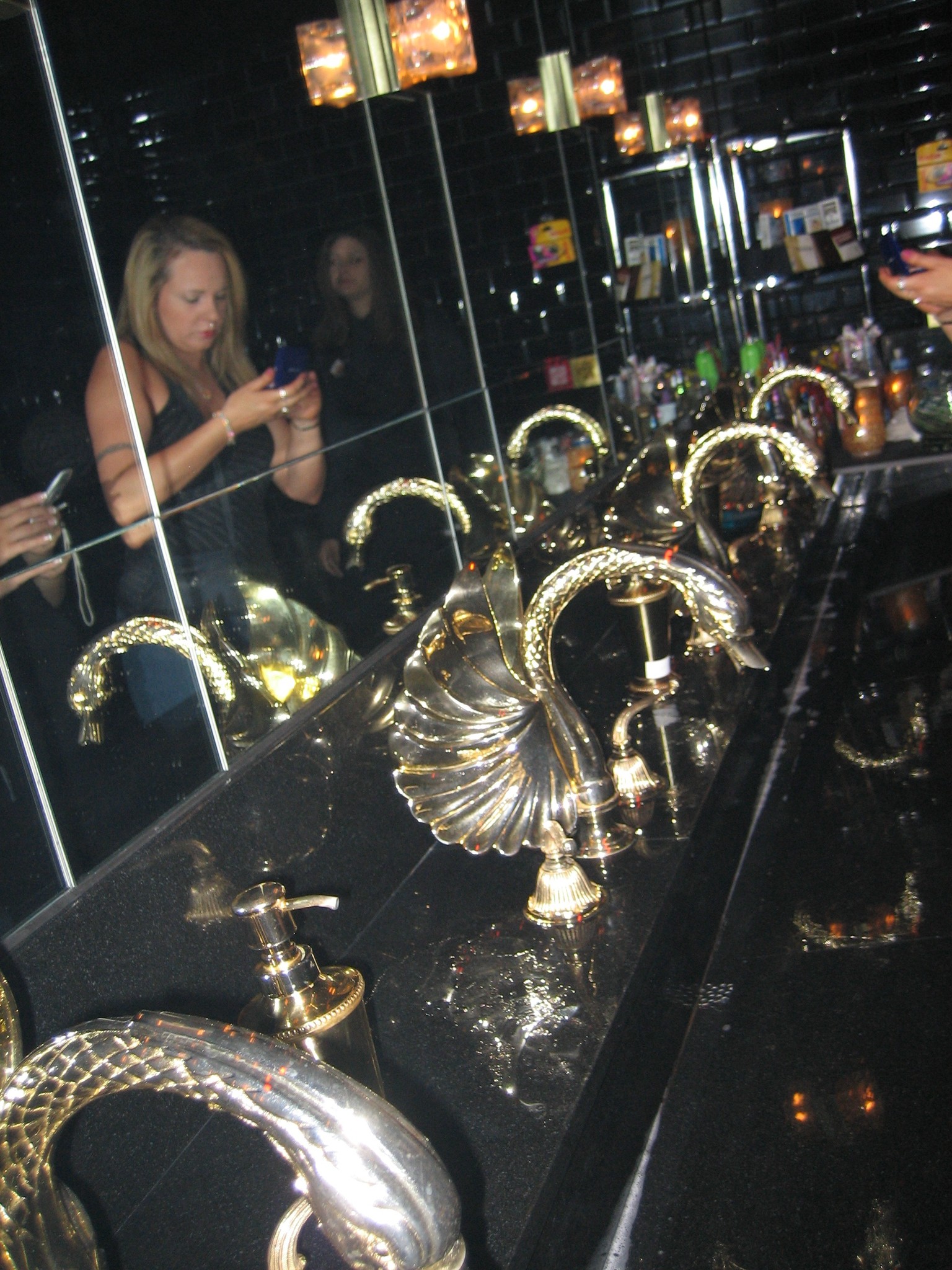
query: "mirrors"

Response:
[2,0,734,945]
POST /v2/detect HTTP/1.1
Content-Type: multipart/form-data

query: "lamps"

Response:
[291,11,646,157]
[381,1,704,160]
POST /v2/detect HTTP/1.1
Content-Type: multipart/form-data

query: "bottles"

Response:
[741,317,950,457]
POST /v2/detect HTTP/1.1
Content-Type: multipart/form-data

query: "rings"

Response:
[278,388,287,400]
[907,291,921,305]
[898,275,906,290]
[281,407,288,415]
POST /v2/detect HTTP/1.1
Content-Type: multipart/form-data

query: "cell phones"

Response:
[29,467,74,524]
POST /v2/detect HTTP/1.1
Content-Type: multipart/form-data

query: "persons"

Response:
[872,231,951,342]
[318,219,402,351]
[0,490,90,618]
[82,214,324,734]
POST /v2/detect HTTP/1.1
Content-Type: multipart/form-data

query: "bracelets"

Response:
[212,408,236,456]
[289,418,321,431]
[939,319,952,327]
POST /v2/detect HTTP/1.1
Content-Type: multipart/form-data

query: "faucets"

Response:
[59,566,363,767]
[505,399,622,489]
[336,452,564,606]
[684,364,863,542]
[2,964,473,1270]
[384,541,775,929]
[598,421,842,608]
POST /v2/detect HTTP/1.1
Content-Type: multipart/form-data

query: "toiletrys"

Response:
[361,561,446,639]
[222,880,391,1119]
